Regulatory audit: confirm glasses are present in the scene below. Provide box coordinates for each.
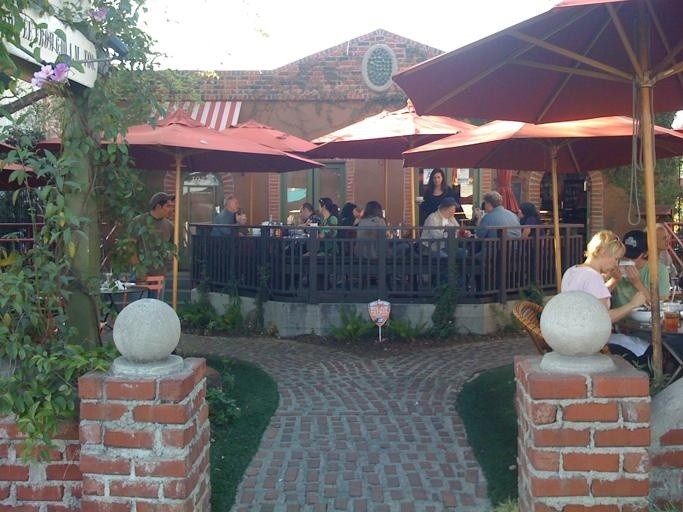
[660,235,670,241]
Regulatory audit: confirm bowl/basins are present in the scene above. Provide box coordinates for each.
[633,306,663,327]
[660,301,682,314]
[122,283,135,288]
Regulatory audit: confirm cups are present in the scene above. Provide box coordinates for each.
[458,228,466,239]
[618,261,634,280]
[663,305,679,335]
[671,276,679,291]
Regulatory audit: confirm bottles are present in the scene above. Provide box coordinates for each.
[268,214,276,236]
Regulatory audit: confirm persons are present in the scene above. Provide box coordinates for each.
[126,192,175,306]
[198,191,538,301]
[422,168,460,213]
[560,221,683,382]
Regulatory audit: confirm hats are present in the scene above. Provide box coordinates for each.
[148,192,175,210]
[621,230,647,258]
[437,197,456,208]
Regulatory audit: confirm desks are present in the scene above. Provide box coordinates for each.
[541,219,563,233]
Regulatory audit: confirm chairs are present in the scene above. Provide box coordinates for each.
[121,275,165,308]
[512,300,609,354]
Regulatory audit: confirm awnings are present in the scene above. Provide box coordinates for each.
[148,101,242,134]
